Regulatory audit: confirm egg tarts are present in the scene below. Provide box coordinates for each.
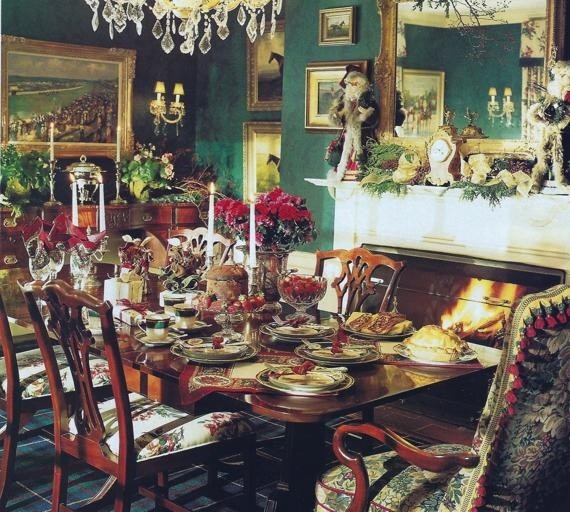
[278,372,335,392]
[275,326,317,335]
[313,347,360,360]
[189,345,246,357]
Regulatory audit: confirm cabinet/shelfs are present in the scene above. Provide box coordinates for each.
[334,182,569,318]
[0,202,209,253]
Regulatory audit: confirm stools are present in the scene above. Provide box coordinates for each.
[0,313,40,359]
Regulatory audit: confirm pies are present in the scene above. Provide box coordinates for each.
[346,312,412,334]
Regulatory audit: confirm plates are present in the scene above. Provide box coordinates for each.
[171,322,212,331]
[255,368,353,394]
[180,336,246,356]
[294,343,382,362]
[340,319,413,335]
[134,327,186,344]
[305,343,372,360]
[171,342,258,361]
[265,321,332,335]
[259,319,337,339]
[392,344,478,363]
[268,367,345,390]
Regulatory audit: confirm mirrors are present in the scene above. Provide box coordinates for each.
[373,1,569,164]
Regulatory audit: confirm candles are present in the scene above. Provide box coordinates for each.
[99,184,107,232]
[72,182,81,227]
[206,195,216,259]
[249,202,257,268]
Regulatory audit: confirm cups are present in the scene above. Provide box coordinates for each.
[164,294,185,313]
[48,251,63,278]
[28,256,50,306]
[174,304,198,328]
[138,314,169,339]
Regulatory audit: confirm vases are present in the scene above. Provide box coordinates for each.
[237,246,293,282]
[9,172,32,202]
[133,179,150,203]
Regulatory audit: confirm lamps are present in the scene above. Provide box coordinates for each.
[483,88,513,130]
[83,1,281,56]
[148,80,186,137]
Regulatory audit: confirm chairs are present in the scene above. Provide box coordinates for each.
[166,227,238,276]
[311,246,406,320]
[0,285,116,510]
[311,287,570,511]
[17,279,256,512]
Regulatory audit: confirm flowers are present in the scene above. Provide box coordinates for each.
[120,142,175,189]
[216,187,315,251]
[0,143,49,195]
[522,19,548,61]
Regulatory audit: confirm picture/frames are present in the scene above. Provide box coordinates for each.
[244,22,286,111]
[304,60,373,133]
[392,66,444,141]
[318,4,356,46]
[1,35,134,161]
[242,121,283,205]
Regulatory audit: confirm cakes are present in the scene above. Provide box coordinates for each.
[404,325,469,361]
[204,264,248,300]
